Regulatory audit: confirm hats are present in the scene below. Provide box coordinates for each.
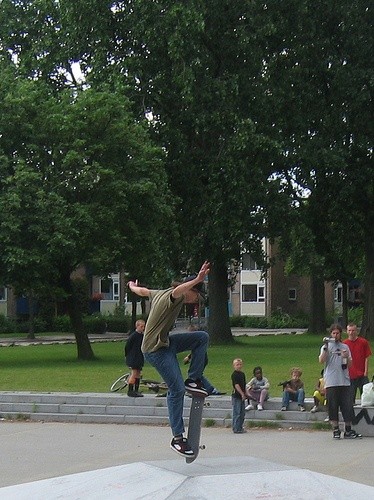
[182,277,206,300]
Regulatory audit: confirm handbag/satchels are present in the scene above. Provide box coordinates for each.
[360,382,374,407]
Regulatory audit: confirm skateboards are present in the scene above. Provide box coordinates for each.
[186,395,210,464]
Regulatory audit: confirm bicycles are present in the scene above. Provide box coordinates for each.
[109,372,170,394]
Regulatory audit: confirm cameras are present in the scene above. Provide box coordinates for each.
[324,337,336,343]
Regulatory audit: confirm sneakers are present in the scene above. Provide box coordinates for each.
[185,378,209,398]
[323,416,330,422]
[241,427,245,430]
[257,404,263,411]
[234,429,246,433]
[244,405,254,411]
[333,428,341,440]
[281,406,286,411]
[310,405,319,413]
[206,386,217,395]
[170,438,195,458]
[135,391,143,397]
[344,430,362,439]
[297,406,306,412]
[127,391,137,397]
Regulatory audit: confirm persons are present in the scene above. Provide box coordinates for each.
[125,320,145,397]
[182,351,217,395]
[232,358,248,433]
[126,260,211,457]
[244,366,270,411]
[281,367,306,411]
[318,323,363,439]
[361,376,374,407]
[342,322,372,406]
[310,369,327,412]
